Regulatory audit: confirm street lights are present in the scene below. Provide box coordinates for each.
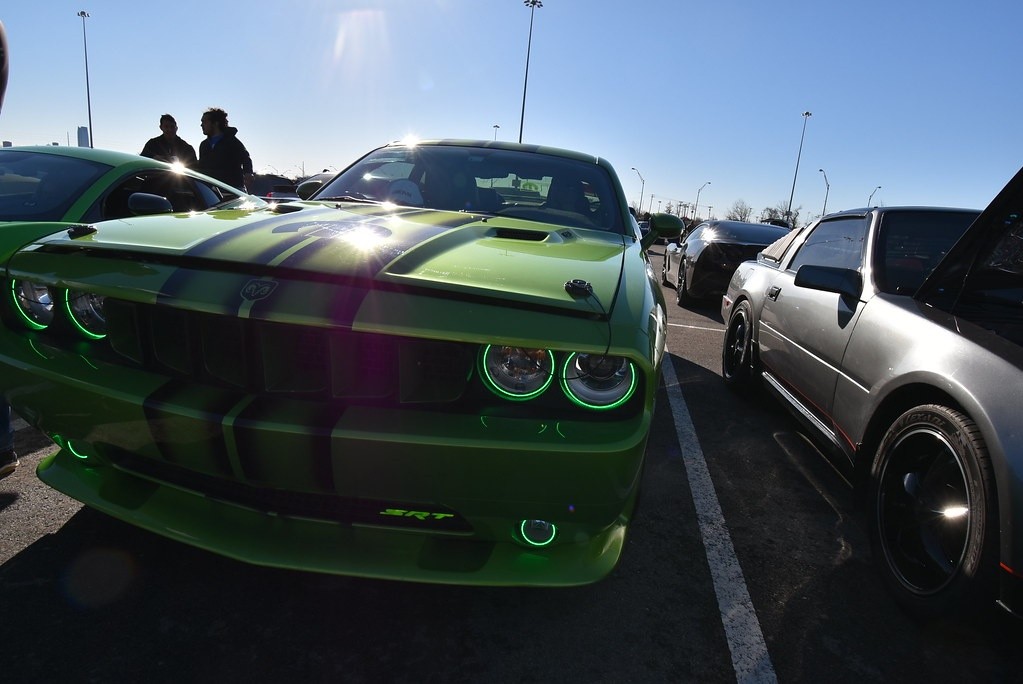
[694,181,711,221]
[519,0,543,143]
[819,169,829,215]
[78,11,94,149]
[632,167,644,215]
[868,186,881,207]
[787,112,812,220]
[491,125,499,188]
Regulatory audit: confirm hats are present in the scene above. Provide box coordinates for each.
[387,179,423,206]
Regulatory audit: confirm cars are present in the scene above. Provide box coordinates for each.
[0,148,269,267]
[0,141,667,594]
[663,220,792,307]
[720,167,1023,618]
[267,173,336,197]
[638,221,649,235]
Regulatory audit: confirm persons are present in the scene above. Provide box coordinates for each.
[199,108,255,194]
[139,114,198,171]
[0,19,18,478]
[686,221,695,233]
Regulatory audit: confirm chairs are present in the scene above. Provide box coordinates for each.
[419,173,583,211]
